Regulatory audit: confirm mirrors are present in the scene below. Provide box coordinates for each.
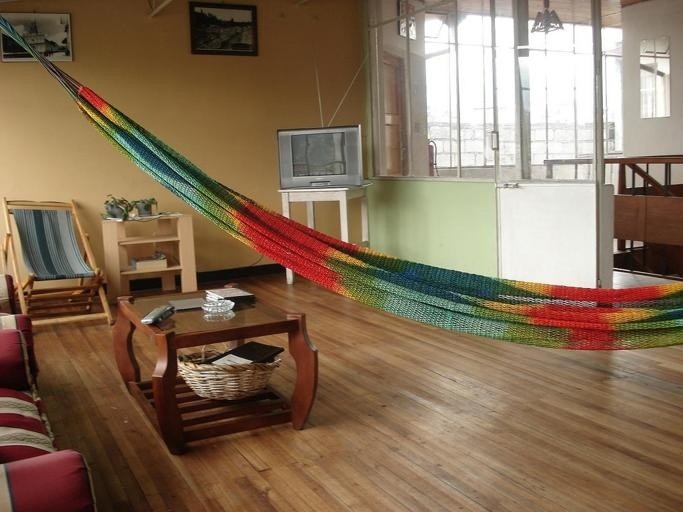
[640,37,671,118]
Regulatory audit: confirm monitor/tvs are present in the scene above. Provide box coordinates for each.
[276,124,364,190]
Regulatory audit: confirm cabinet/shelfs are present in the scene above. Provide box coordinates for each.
[101,212,199,304]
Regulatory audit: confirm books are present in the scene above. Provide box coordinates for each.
[204,287,255,303]
[203,339,283,366]
[129,250,168,273]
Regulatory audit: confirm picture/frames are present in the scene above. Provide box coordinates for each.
[0,12,73,62]
[396,0,417,41]
[189,0,259,57]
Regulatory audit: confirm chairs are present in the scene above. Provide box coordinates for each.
[1,197,115,334]
[0,312,99,511]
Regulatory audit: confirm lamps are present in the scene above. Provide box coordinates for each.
[530,0,565,36]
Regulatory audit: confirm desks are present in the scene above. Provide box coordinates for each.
[276,181,375,285]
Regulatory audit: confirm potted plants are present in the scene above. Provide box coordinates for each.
[103,193,134,220]
[128,196,160,217]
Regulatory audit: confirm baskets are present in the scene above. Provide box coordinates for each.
[177,341,282,401]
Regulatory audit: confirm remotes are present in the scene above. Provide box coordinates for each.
[140,305,173,325]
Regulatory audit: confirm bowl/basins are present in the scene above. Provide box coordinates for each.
[203,315,235,321]
[202,301,234,313]
